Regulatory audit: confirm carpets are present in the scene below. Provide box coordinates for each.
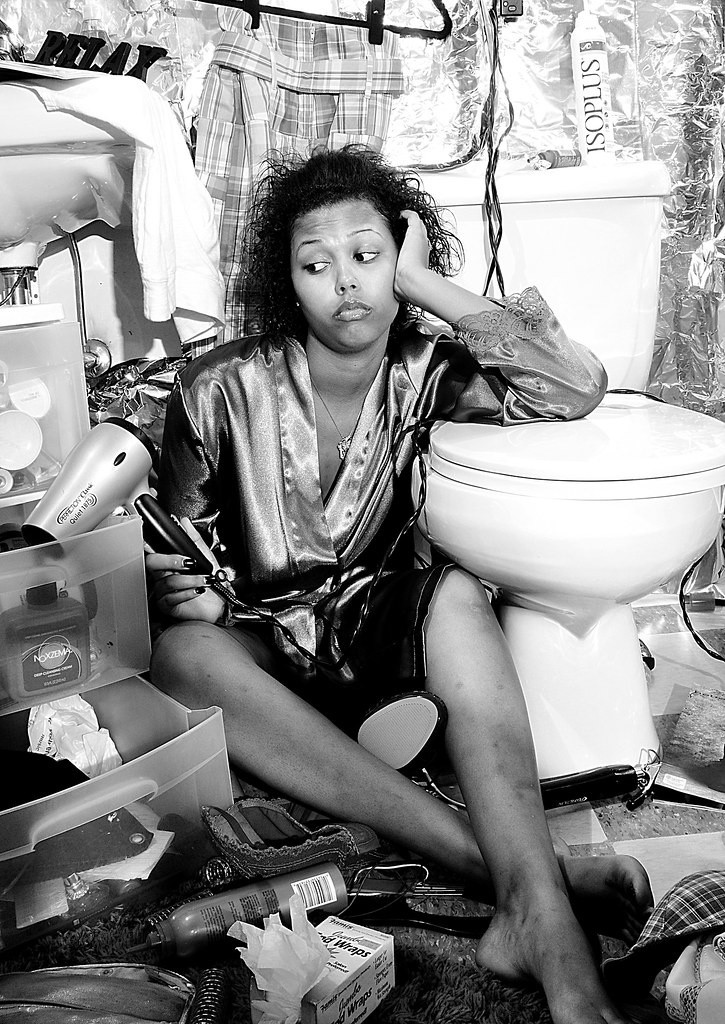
[1,885,670,1024]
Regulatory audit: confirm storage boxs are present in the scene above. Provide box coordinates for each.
[250,915,395,1024]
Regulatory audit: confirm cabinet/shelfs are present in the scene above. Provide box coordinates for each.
[0,302,236,953]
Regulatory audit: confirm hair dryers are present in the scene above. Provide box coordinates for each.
[21,417,213,575]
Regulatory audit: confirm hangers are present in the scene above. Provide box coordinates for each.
[197,0,453,47]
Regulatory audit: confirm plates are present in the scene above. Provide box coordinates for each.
[0,410,43,470]
[7,378,51,418]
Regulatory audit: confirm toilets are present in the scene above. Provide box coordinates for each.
[412,394,725,769]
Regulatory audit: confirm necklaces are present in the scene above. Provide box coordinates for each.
[310,377,362,460]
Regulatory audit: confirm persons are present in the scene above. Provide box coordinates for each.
[146,145,654,1024]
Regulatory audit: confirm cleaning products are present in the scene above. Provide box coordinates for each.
[570,10,614,160]
